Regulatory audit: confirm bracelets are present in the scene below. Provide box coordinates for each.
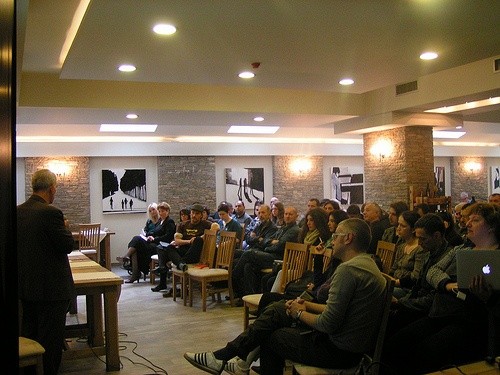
[296,309,303,319]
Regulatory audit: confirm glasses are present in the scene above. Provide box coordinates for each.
[331,233,347,240]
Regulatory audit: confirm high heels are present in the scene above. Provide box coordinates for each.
[125,272,142,283]
[115,256,131,264]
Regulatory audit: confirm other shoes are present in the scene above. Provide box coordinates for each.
[251,366,262,374]
[150,285,167,292]
[162,287,181,298]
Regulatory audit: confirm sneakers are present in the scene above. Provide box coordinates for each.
[224,360,250,375]
[184,350,226,375]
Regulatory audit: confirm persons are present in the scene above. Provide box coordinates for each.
[205,196,500,306]
[116,202,174,285]
[384,200,499,375]
[15,168,77,373]
[184,218,387,375]
[178,208,191,222]
[166,203,214,273]
[389,214,460,329]
[149,208,191,292]
[251,242,343,375]
[382,210,426,295]
[121,203,161,269]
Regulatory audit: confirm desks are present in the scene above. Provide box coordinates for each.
[65,250,124,372]
[72,232,115,271]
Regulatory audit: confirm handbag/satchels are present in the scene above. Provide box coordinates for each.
[284,270,318,297]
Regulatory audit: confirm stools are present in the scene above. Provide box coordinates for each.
[18,336,46,375]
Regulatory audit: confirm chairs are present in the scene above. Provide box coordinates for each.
[78,224,101,263]
[242,240,395,375]
[151,230,236,312]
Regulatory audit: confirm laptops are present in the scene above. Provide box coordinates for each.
[455,249,500,291]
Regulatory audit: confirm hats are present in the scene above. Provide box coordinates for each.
[217,200,230,213]
[190,204,204,212]
